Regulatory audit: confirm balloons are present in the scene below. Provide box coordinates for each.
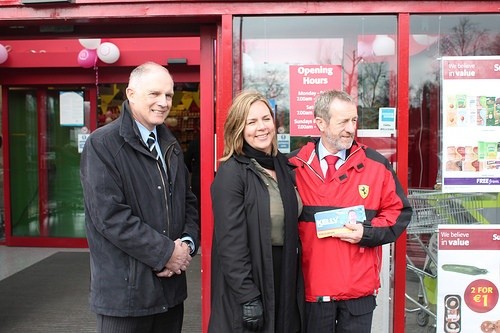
[78,39,101,51]
[0,44,8,64]
[78,49,97,68]
[98,42,120,64]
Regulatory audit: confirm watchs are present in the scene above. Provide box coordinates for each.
[182,240,194,255]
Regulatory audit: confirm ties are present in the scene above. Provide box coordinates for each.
[324,155,339,182]
[147,132,171,211]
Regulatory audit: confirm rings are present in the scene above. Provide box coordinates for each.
[169,272,173,274]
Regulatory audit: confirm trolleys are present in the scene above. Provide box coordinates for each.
[406,188,485,332]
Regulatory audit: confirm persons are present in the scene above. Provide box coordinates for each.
[207,92,303,333]
[285,91,413,333]
[342,210,361,227]
[79,62,200,333]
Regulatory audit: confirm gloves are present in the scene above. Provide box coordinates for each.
[242,298,264,333]
